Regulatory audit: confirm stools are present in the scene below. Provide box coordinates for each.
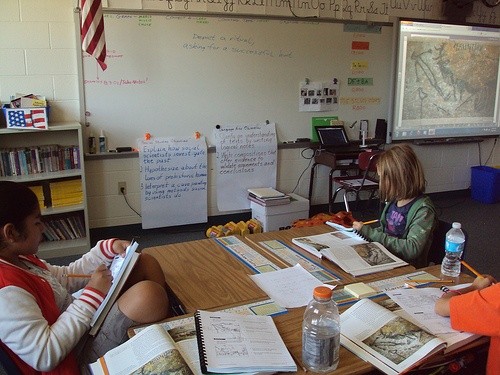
[251,193,309,232]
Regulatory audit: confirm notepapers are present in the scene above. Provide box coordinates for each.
[344,282,377,299]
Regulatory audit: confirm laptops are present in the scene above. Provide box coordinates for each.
[315,125,365,154]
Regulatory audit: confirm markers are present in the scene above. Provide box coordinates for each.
[282,141,297,143]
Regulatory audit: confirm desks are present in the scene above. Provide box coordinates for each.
[128,220,491,375]
[308,145,386,214]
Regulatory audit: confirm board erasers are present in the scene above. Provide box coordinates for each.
[297,138,310,142]
[116,147,132,152]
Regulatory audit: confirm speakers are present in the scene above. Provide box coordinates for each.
[376,119,387,144]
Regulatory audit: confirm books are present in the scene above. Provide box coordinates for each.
[30,180,82,209]
[196,309,297,375]
[248,188,291,206]
[89,317,200,375]
[72,238,141,336]
[335,282,500,375]
[0,144,80,177]
[41,215,85,240]
[293,231,409,278]
[10,94,46,109]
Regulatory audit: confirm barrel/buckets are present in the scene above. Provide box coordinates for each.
[470,165,500,204]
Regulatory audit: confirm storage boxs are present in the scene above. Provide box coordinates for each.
[2,104,50,128]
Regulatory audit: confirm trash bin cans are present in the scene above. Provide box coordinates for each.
[470,166,500,203]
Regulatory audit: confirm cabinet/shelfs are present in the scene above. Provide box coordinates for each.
[0,121,90,259]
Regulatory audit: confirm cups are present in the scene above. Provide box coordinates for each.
[441,222,466,277]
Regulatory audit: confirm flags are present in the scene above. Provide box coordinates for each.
[78,0,108,71]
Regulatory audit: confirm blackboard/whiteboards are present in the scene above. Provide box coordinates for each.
[74,5,395,160]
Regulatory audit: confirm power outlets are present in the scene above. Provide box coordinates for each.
[118,181,127,196]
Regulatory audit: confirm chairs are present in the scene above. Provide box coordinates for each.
[432,220,467,265]
[331,151,384,220]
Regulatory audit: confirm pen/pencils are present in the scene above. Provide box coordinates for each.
[364,219,379,225]
[458,257,485,279]
[405,280,454,282]
[62,274,91,277]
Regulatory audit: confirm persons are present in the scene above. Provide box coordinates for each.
[352,143,436,263]
[434,274,500,375]
[0,181,169,375]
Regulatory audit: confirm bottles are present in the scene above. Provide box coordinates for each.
[301,285,341,374]
[88,133,96,153]
[99,128,106,153]
